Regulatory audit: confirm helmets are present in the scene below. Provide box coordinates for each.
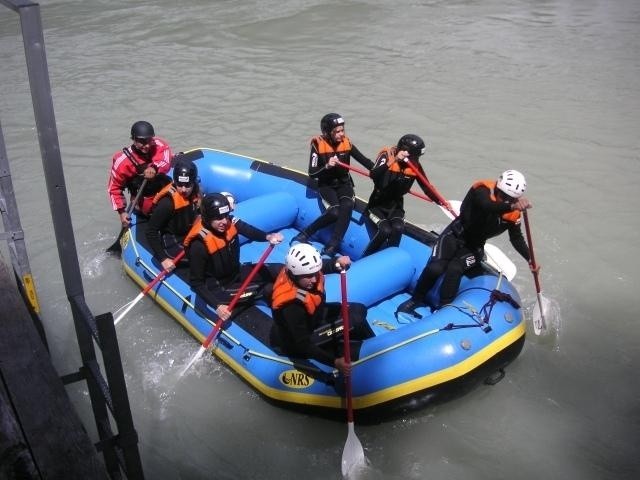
[397,134,426,157]
[496,169,527,199]
[131,121,156,139]
[200,193,232,219]
[286,244,323,276]
[173,159,198,186]
[321,113,345,132]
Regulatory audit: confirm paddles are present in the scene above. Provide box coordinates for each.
[334,155,463,220]
[112,249,184,326]
[182,238,277,375]
[105,174,148,257]
[404,157,517,282]
[335,263,367,479]
[523,207,551,336]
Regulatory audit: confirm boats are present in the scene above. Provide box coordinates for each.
[123,146,525,429]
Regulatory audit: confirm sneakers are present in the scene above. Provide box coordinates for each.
[397,297,424,314]
[288,230,313,247]
[321,247,336,259]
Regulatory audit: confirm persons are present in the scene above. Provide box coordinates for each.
[146,160,205,274]
[272,243,375,385]
[189,192,285,322]
[107,120,173,229]
[289,112,375,258]
[394,170,541,313]
[362,133,453,256]
